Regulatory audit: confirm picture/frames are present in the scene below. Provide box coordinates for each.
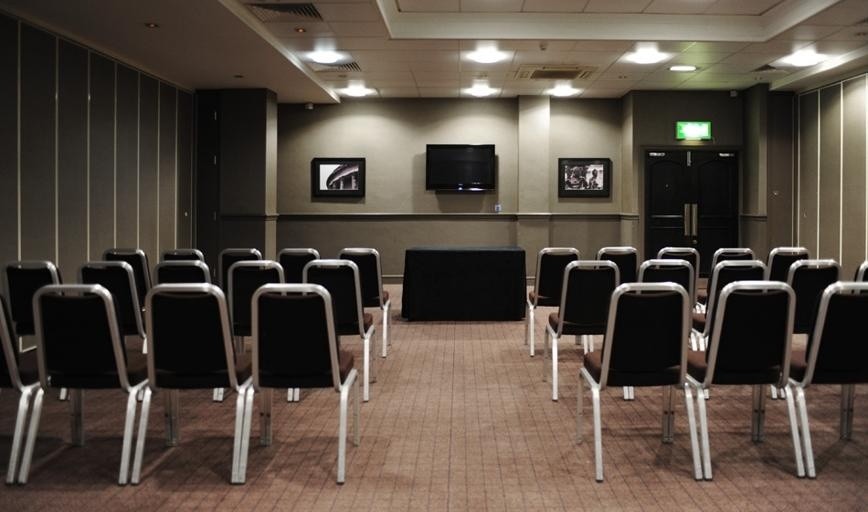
[557,157,611,198]
[311,156,366,199]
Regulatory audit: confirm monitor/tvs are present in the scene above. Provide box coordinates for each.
[426,144,495,191]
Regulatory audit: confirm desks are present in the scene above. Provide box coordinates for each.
[401,244,527,321]
